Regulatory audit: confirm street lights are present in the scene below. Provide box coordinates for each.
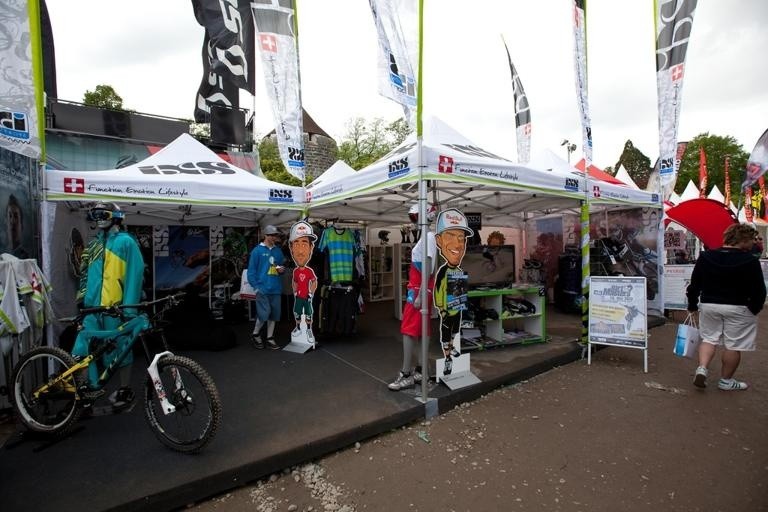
[559,139,576,168]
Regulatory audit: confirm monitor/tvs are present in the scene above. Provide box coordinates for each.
[461,245,515,290]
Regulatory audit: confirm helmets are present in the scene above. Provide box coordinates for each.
[378,231,390,242]
[95,202,120,223]
[522,259,543,269]
[407,203,435,222]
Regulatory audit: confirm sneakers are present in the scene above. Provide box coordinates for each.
[717,379,747,391]
[443,359,451,374]
[250,334,264,350]
[306,329,313,343]
[112,386,136,413]
[413,366,429,384]
[387,370,414,391]
[449,347,459,357]
[692,368,707,388]
[265,337,280,350]
[291,327,301,336]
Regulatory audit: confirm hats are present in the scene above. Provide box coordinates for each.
[290,222,315,242]
[263,225,280,234]
[436,209,474,237]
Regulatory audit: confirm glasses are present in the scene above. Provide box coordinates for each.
[409,214,417,223]
[92,210,124,220]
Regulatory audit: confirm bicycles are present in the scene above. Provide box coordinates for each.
[8,292,223,458]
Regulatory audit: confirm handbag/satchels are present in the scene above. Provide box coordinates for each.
[672,313,699,359]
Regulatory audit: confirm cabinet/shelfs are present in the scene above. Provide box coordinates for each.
[363,245,395,302]
[453,287,546,354]
[395,243,415,320]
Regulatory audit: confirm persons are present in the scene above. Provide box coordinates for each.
[530,232,560,305]
[288,221,318,343]
[487,231,506,245]
[1,193,30,259]
[482,244,493,262]
[685,223,767,390]
[432,207,475,374]
[72,201,147,411]
[247,225,288,349]
[387,204,435,392]
